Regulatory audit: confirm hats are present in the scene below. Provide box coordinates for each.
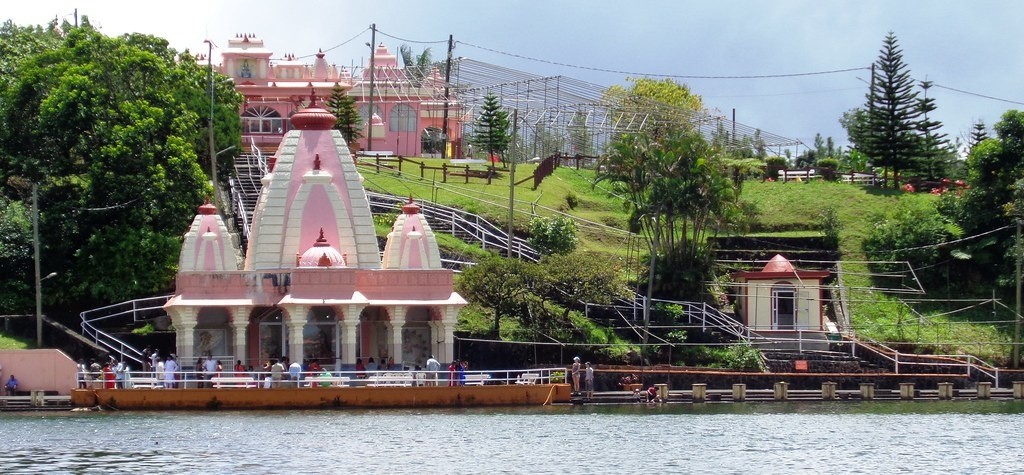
[457,359,461,362]
[573,357,580,360]
[90,363,101,371]
[636,389,639,392]
[585,362,590,364]
[453,360,457,363]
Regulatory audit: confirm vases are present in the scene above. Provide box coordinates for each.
[623,384,644,390]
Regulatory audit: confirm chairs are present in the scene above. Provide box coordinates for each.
[383,374,413,387]
[416,373,426,386]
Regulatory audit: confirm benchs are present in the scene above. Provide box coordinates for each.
[211,377,256,389]
[463,375,491,386]
[10,391,58,396]
[367,376,412,388]
[515,373,539,385]
[115,378,163,389]
[304,377,350,388]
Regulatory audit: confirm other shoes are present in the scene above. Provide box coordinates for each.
[646,400,649,403]
[4,392,8,396]
[650,400,655,403]
[572,392,580,396]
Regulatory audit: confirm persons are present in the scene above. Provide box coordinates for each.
[196,354,223,388]
[585,362,594,398]
[426,355,440,386]
[356,357,386,378]
[448,359,465,386]
[77,358,131,390]
[235,357,332,388]
[4,375,18,396]
[572,356,581,394]
[142,345,180,388]
[646,387,659,402]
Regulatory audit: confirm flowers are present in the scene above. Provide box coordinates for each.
[619,374,641,386]
